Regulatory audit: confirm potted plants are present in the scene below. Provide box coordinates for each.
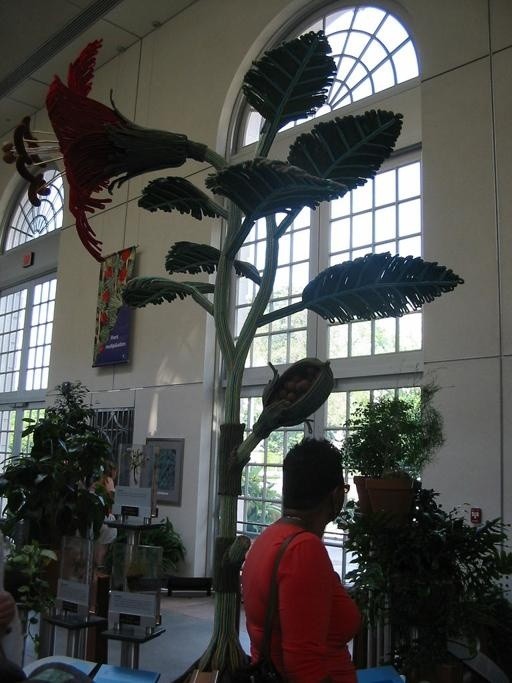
[366,391,447,526]
[338,519,510,683]
[105,516,186,591]
[339,403,372,512]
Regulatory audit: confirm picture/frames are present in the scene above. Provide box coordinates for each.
[140,438,184,506]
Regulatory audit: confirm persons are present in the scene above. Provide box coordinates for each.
[241,439,363,683]
[89,461,118,570]
[0,532,29,682]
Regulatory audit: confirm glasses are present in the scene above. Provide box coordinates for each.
[339,484,349,493]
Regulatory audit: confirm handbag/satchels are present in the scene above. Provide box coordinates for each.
[235,656,281,683]
